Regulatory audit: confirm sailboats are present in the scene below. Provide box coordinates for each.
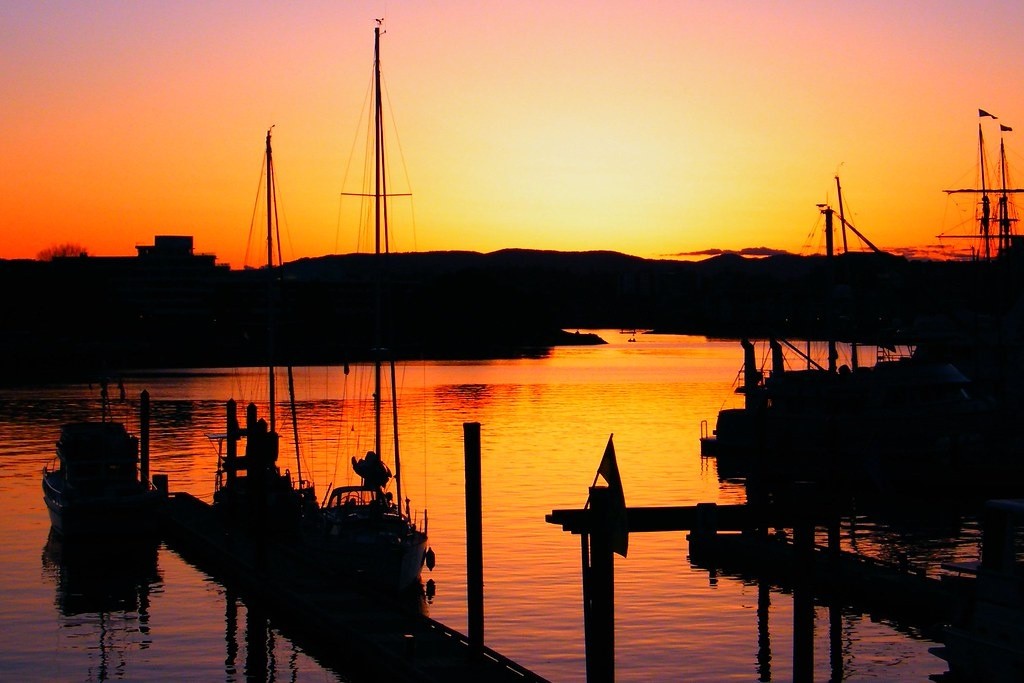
[297,18,430,599]
[204,122,318,546]
[697,107,1024,489]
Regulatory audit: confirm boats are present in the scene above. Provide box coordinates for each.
[40,371,161,545]
